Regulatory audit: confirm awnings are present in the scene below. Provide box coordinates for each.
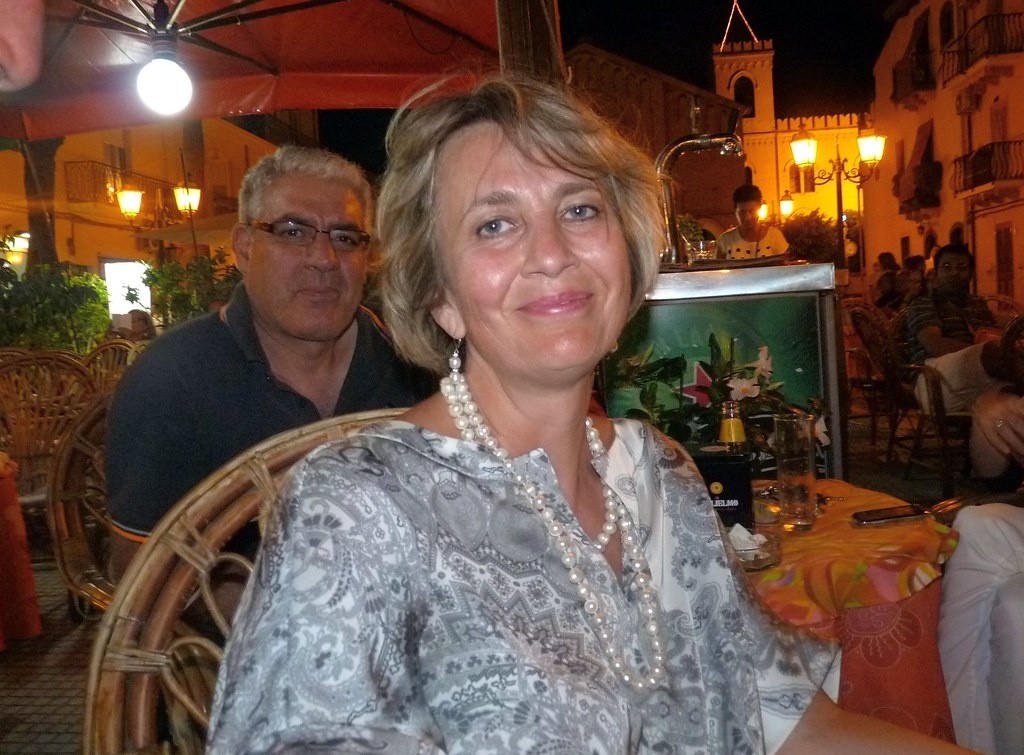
[898,7,931,99]
[899,120,934,203]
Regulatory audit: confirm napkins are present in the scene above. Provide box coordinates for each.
[728,522,771,562]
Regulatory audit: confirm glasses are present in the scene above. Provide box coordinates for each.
[248,218,372,253]
[937,263,972,272]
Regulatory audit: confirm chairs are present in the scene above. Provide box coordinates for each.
[840,292,1024,498]
[45,389,219,755]
[84,405,413,755]
[0,339,152,554]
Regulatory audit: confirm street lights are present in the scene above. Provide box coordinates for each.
[115,166,203,331]
[788,111,888,287]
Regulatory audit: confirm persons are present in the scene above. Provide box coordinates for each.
[871,252,936,311]
[206,66,976,754]
[903,246,1024,414]
[209,300,227,310]
[713,184,790,262]
[967,392,1024,494]
[105,145,439,647]
[118,310,156,341]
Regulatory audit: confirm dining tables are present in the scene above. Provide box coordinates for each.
[724,478,960,746]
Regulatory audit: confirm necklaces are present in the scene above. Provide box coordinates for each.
[439,371,666,696]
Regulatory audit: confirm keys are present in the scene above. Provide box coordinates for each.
[817,493,846,503]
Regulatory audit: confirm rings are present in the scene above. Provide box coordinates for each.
[994,420,1004,430]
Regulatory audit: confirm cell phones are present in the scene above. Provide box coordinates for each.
[853,505,925,526]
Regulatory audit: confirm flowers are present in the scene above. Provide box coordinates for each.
[694,332,786,406]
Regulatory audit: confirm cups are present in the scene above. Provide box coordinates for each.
[686,240,718,262]
[774,413,816,532]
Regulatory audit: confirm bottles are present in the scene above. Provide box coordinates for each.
[719,400,749,453]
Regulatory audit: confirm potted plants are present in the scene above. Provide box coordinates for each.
[676,215,718,260]
